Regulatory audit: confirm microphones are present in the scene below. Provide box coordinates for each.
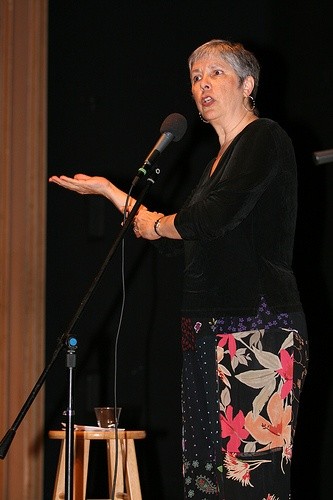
[132,113,188,184]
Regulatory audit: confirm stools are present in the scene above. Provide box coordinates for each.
[48,430,146,500]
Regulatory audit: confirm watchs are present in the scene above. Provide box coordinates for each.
[155,217,164,238]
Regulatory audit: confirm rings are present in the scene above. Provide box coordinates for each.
[133,227,139,232]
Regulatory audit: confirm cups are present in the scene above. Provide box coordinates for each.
[93,407,121,429]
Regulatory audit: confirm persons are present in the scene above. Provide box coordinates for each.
[49,40,309,500]
